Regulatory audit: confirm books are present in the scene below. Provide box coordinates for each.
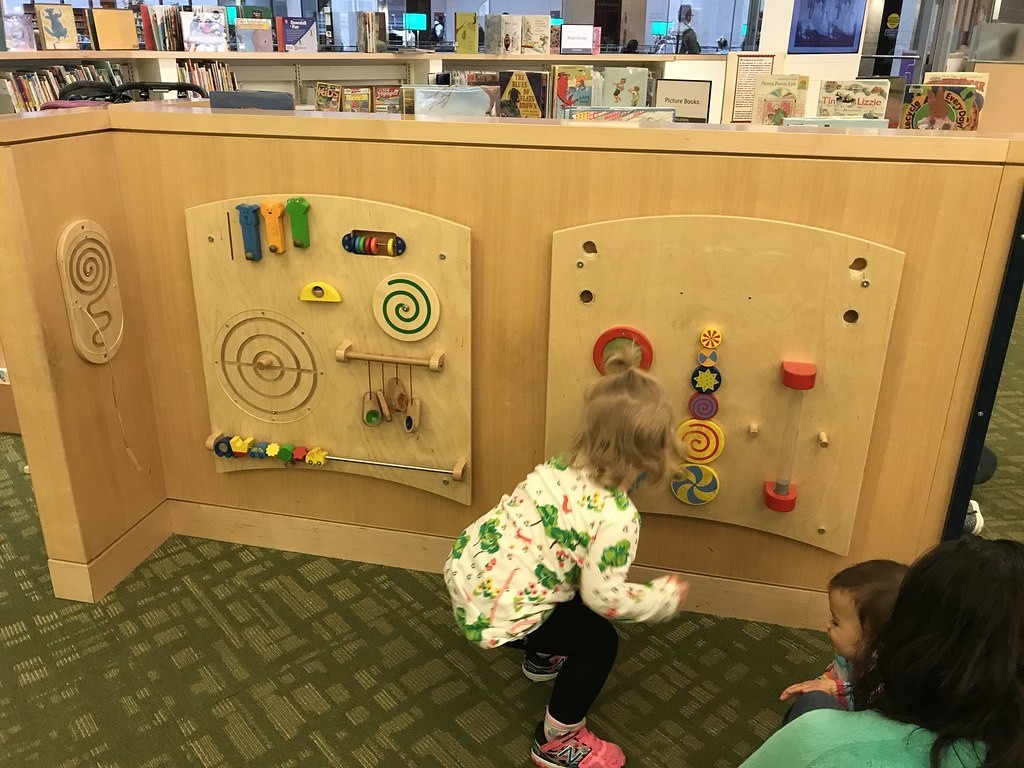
[1,1,1022,129]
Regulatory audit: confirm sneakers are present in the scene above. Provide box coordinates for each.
[522,647,569,685]
[529,719,626,768]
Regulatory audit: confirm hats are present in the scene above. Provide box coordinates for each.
[678,4,694,18]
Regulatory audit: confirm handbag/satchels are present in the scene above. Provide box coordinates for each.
[427,23,443,44]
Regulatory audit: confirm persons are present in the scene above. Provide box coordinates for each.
[442,333,691,768]
[779,558,911,711]
[738,534,1024,768]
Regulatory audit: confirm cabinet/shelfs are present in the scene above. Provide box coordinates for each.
[0,45,733,117]
